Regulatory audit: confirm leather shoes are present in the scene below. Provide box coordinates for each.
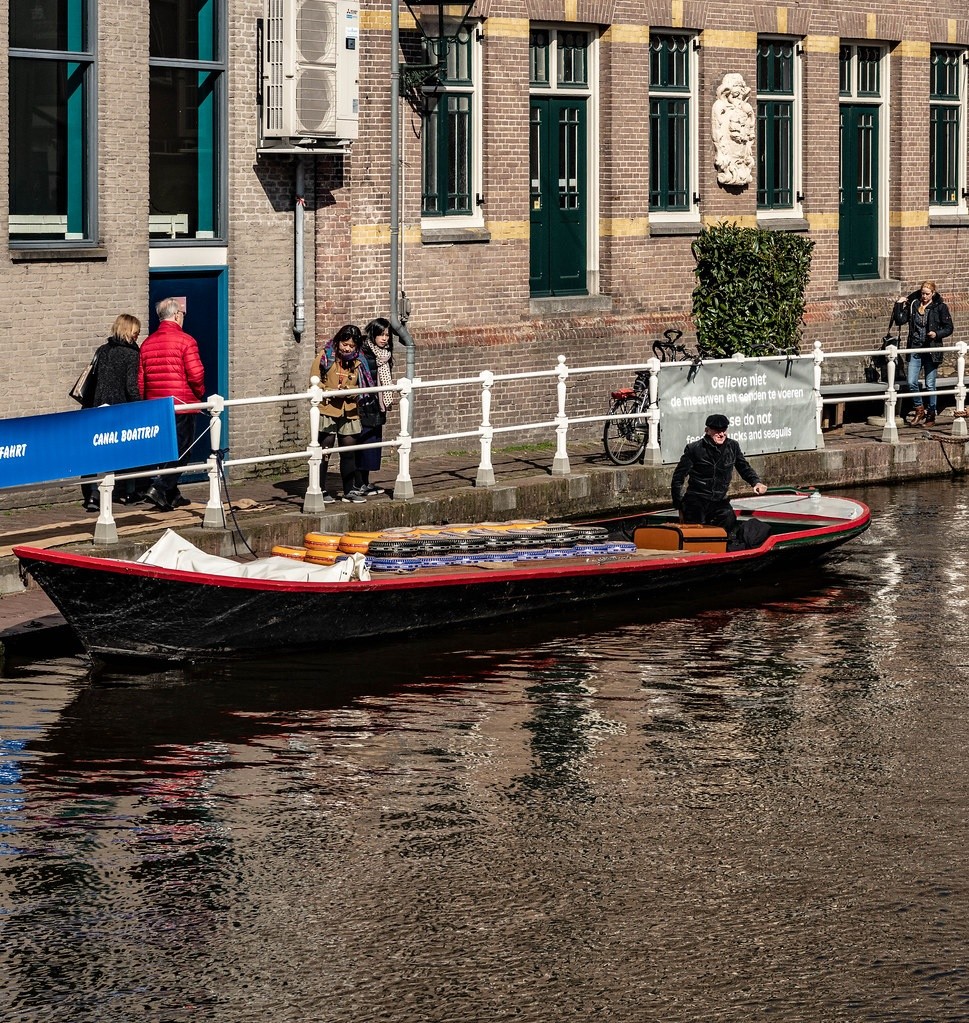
[125,492,147,505]
[166,495,191,507]
[87,496,100,509]
[146,486,173,510]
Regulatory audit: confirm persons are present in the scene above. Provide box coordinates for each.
[86,314,147,509]
[894,281,954,429]
[671,414,768,552]
[308,317,394,503]
[137,297,205,513]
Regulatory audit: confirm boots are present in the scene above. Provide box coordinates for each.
[911,404,925,425]
[921,410,936,427]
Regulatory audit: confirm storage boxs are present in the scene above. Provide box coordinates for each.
[631,524,729,552]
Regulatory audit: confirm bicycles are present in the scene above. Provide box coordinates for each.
[602,329,796,466]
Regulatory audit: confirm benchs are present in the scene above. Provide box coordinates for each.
[820,377,969,429]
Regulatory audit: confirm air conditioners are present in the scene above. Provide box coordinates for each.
[264,0,359,140]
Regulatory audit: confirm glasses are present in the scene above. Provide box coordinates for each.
[177,310,185,314]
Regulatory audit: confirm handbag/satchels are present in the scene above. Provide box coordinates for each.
[880,334,901,349]
[69,345,104,406]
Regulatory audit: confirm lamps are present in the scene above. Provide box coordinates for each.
[396,0,477,96]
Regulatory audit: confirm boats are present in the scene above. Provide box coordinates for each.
[11,487,873,671]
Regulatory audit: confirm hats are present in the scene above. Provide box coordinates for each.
[705,414,729,430]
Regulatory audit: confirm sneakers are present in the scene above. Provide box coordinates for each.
[323,490,336,504]
[341,492,367,503]
[368,483,385,493]
[350,484,378,495]
